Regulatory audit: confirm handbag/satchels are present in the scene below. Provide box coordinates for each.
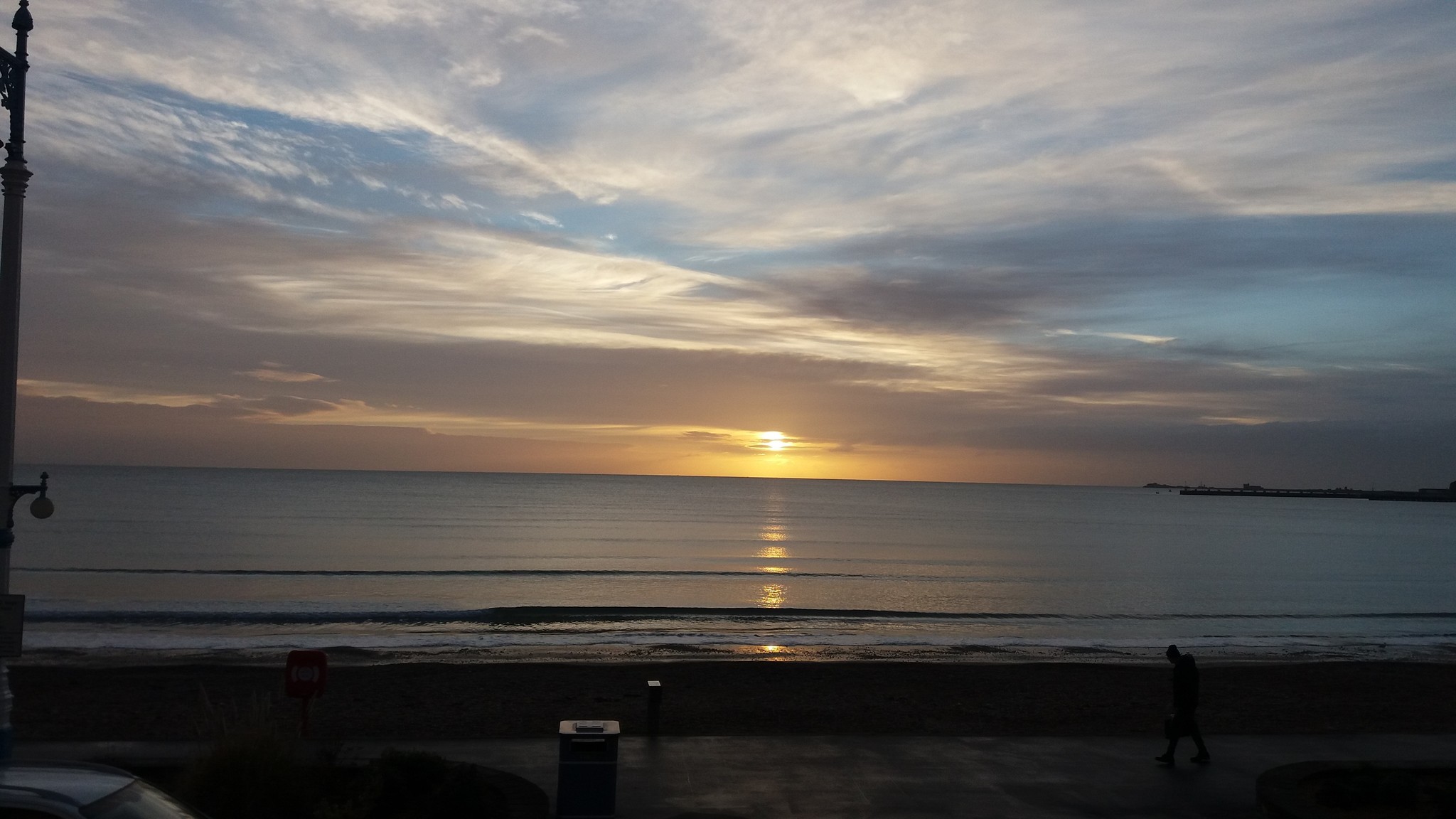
[1163,719,1175,739]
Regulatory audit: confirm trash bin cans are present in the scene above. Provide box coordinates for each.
[0,594,25,658]
[557,720,620,819]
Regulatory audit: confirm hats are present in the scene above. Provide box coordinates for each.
[1166,645,1180,658]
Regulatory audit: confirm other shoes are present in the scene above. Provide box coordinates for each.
[1190,754,1210,762]
[1154,754,1174,763]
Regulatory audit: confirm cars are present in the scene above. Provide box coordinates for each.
[0,760,198,818]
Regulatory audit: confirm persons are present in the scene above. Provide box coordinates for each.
[1155,644,1210,763]
